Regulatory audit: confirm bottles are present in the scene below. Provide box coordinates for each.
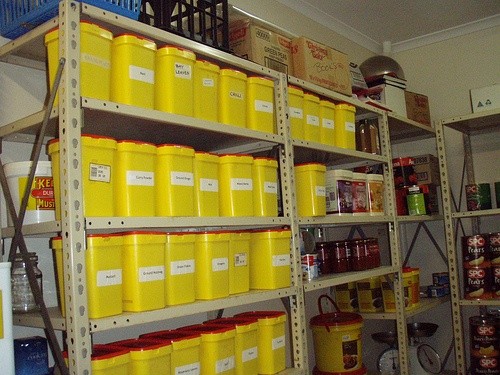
[10,250,44,313]
[318,237,380,275]
[408,186,426,215]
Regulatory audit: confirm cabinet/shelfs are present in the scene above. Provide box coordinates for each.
[0,0,435,375]
[434,108,500,375]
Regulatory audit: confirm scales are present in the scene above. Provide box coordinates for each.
[408,322,448,375]
[372,331,399,375]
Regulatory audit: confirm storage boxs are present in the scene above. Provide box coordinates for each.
[336,266,449,312]
[469,83,500,113]
[411,153,440,186]
[404,90,430,124]
[292,33,352,97]
[138,0,234,54]
[369,83,407,118]
[155,44,197,117]
[325,170,354,216]
[335,101,357,150]
[218,19,294,76]
[170,57,336,375]
[44,18,170,375]
[349,61,369,96]
[352,173,385,217]
[0,0,141,40]
[421,184,439,214]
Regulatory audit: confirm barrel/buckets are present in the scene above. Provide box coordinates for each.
[309,295,366,375]
[3,161,58,224]
